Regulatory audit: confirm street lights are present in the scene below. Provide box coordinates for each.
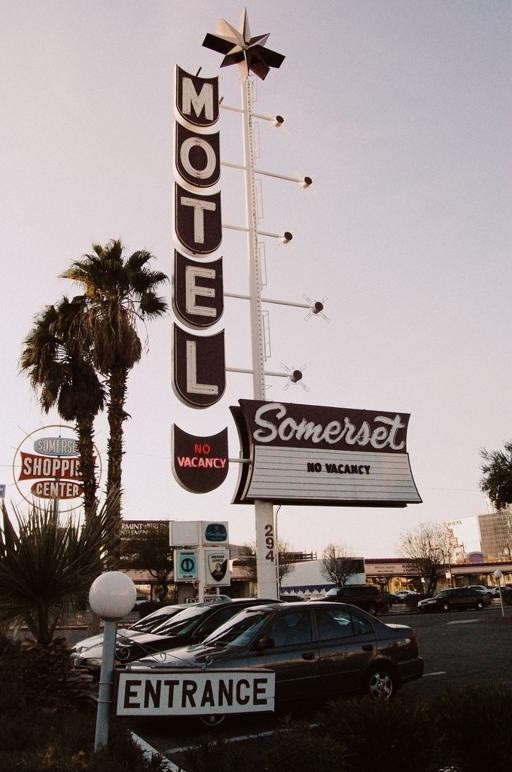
[494,569,504,616]
[88,571,137,752]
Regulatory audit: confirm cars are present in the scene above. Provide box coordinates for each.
[418,584,512,613]
